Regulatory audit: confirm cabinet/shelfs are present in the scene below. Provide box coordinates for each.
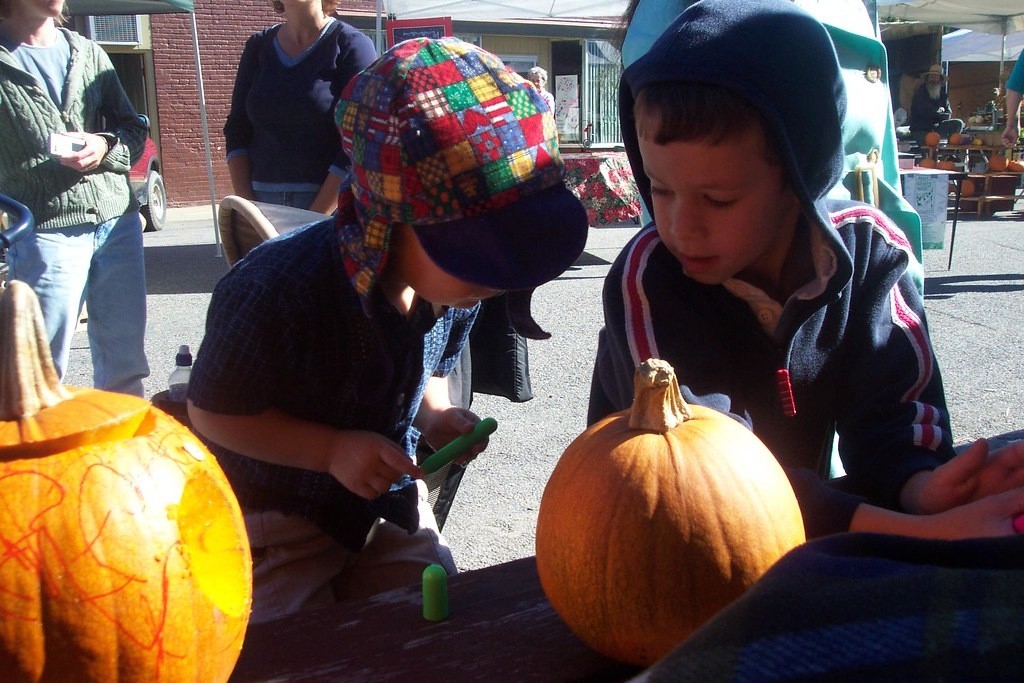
[920,115,1024,222]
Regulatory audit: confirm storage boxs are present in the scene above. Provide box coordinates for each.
[976,131,1003,146]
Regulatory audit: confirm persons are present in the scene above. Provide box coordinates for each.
[0,2,153,410]
[586,0,1024,549]
[524,65,555,122]
[1001,45,1024,150]
[222,0,380,216]
[184,35,590,635]
[618,0,924,303]
[909,64,964,159]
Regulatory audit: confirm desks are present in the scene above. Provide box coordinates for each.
[560,153,642,227]
[228,440,979,683]
[899,165,970,271]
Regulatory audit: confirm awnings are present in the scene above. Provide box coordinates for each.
[383,2,632,38]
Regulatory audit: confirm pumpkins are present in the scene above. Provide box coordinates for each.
[919,129,1024,197]
[532,356,805,667]
[0,278,253,683]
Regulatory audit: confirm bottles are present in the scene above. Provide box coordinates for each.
[168,345,192,401]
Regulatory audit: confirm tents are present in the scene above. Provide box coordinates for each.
[875,0,1024,72]
[67,0,224,260]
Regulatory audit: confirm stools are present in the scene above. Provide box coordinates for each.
[959,175,1017,212]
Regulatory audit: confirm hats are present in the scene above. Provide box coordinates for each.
[332,36,590,303]
[920,64,950,83]
